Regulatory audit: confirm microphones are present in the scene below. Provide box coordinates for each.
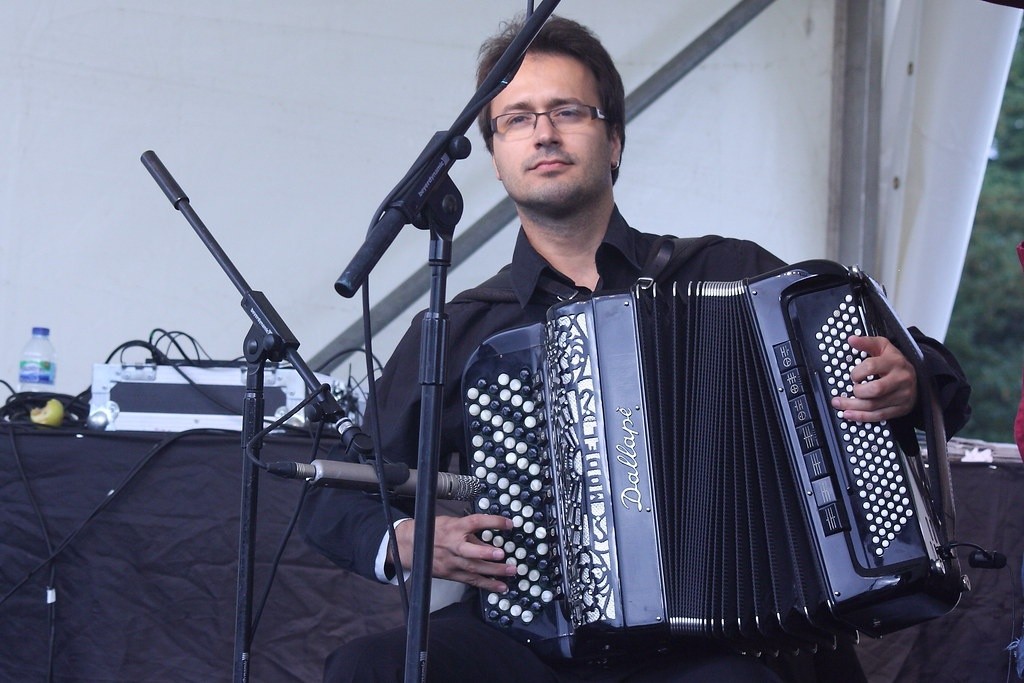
[265,458,481,503]
[944,542,1008,570]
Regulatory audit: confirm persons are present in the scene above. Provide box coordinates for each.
[297,14,973,683]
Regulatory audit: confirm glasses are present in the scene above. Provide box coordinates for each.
[491,103,610,137]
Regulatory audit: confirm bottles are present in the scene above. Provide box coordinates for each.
[18,327,56,393]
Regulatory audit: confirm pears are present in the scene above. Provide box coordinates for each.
[30,398,63,426]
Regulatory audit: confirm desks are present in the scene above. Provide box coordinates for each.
[0,421,1024,683]
